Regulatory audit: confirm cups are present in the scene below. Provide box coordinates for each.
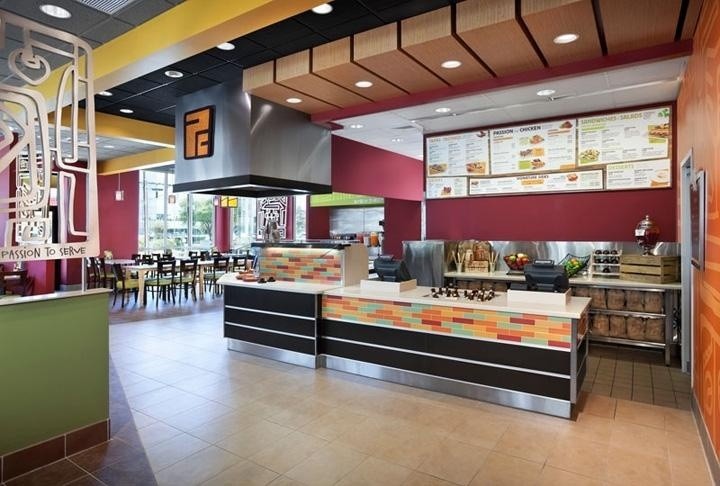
[456,263,463,271]
[490,263,495,274]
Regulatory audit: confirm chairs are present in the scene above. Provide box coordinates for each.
[0,270,28,296]
[89,248,259,308]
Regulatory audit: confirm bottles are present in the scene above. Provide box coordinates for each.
[357,232,379,247]
[635,214,659,256]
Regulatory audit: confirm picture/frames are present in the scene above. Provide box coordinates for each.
[689,171,706,272]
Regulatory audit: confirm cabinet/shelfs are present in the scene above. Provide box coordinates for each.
[590,248,623,279]
[451,276,674,367]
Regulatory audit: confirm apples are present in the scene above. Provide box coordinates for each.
[505,256,528,270]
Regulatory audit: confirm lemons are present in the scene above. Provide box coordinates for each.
[563,258,584,275]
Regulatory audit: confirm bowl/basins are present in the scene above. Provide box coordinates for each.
[504,257,532,271]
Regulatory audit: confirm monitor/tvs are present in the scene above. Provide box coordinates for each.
[524,263,569,293]
